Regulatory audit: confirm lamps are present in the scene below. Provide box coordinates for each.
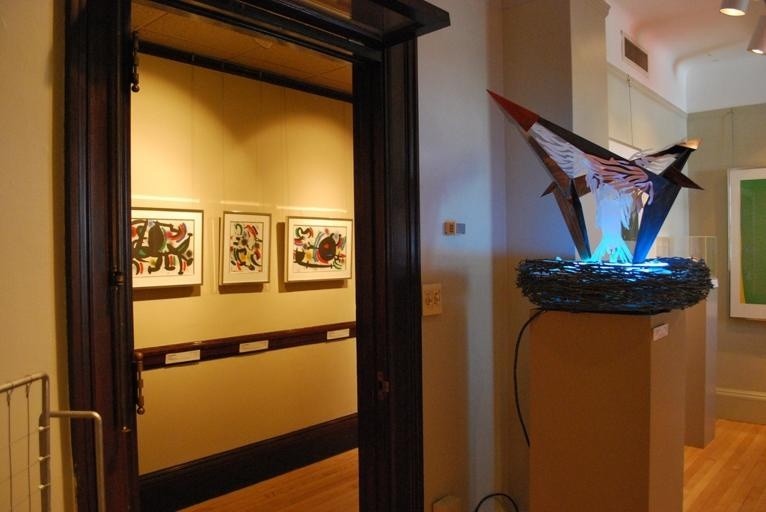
[746,13,765,58]
[716,0,751,19]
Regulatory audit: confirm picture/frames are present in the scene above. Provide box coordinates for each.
[725,167,766,324]
[217,209,276,289]
[128,205,209,291]
[282,215,354,288]
